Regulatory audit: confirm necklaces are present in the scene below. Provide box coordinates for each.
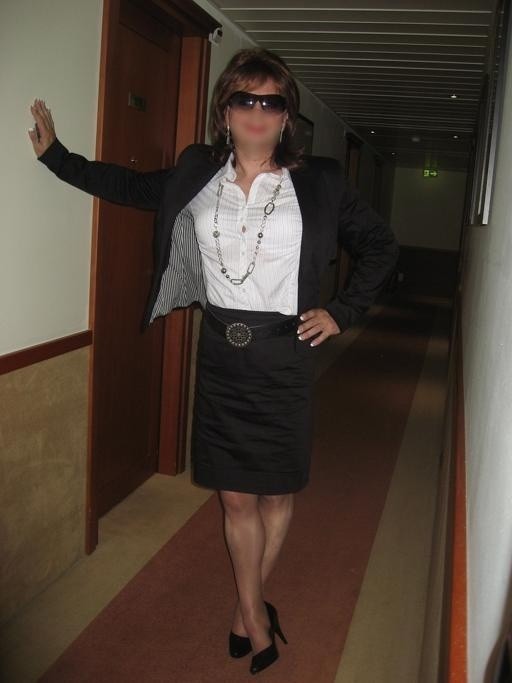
[213,166,284,285]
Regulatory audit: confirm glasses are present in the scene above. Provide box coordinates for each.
[227,91,288,116]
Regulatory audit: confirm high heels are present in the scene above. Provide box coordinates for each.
[229,600,289,675]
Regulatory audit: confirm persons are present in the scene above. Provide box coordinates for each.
[28,46,400,674]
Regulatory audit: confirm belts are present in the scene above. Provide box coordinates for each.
[205,304,297,349]
